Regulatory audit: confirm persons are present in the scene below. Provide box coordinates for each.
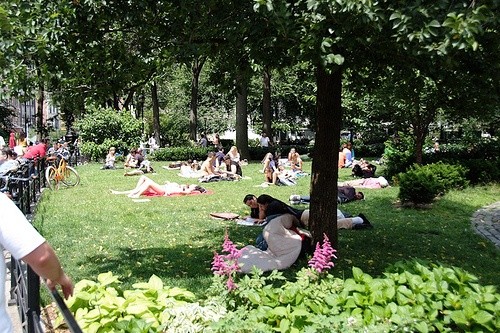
[259,132,274,147]
[433,140,441,152]
[0,190,74,333]
[0,130,80,179]
[101,131,242,199]
[261,147,308,187]
[235,193,372,271]
[338,142,389,188]
[289,185,364,205]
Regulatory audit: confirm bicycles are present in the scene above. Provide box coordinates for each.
[45,139,80,191]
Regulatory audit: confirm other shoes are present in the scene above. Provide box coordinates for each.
[289,195,301,201]
[290,201,300,205]
[358,213,373,228]
